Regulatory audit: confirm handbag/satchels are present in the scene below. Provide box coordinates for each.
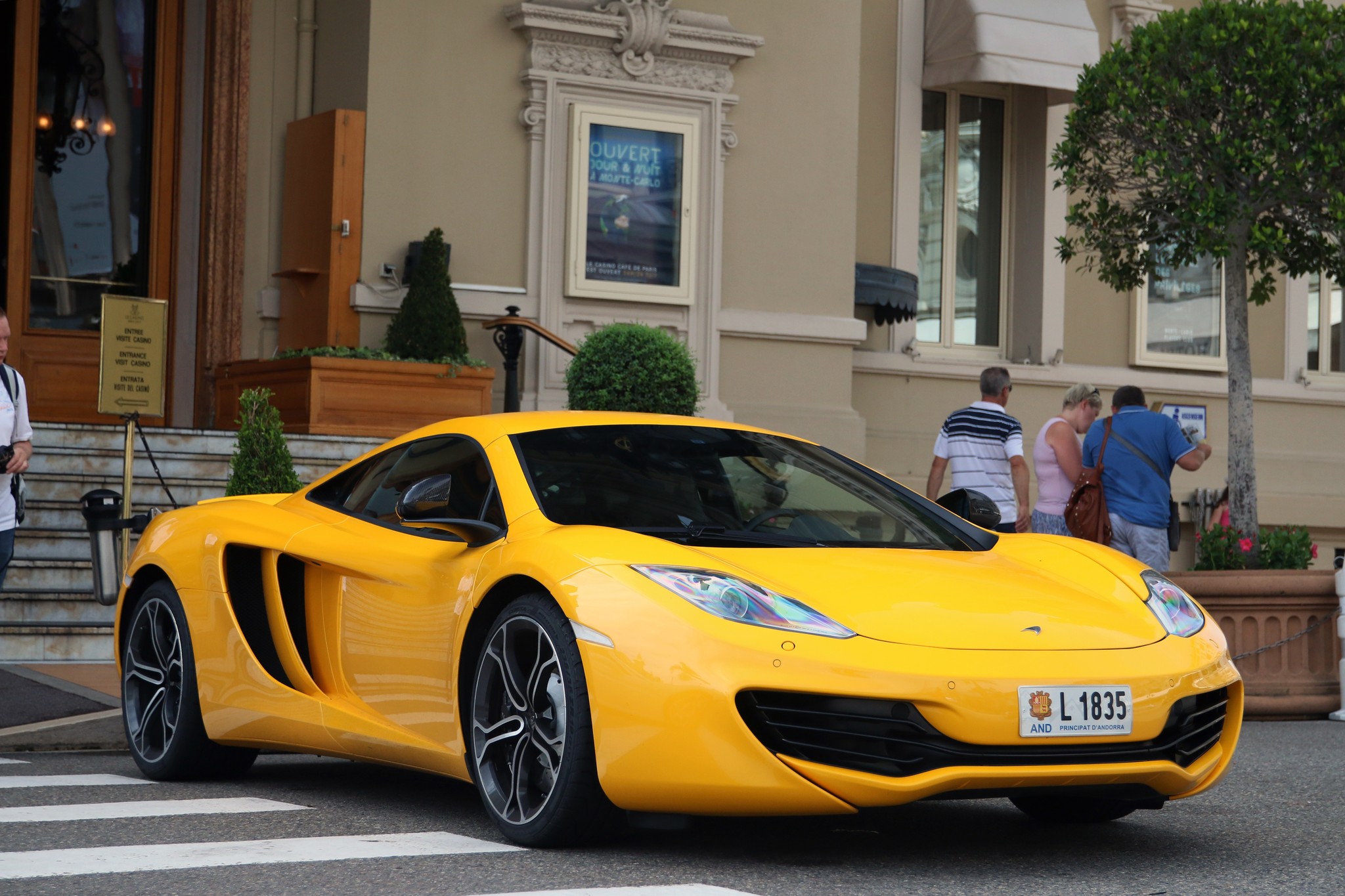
[1168,500,1182,552]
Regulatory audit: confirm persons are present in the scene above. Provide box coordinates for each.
[0,310,34,595]
[926,366,1030,535]
[1032,384,1103,538]
[1081,385,1212,573]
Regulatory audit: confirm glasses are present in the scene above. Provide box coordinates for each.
[1089,387,1100,396]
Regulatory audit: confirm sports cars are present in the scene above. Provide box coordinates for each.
[111,407,1248,845]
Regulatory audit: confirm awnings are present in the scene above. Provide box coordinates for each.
[854,262,919,326]
[923,1,1102,106]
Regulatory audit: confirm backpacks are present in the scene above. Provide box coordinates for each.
[1064,415,1114,547]
